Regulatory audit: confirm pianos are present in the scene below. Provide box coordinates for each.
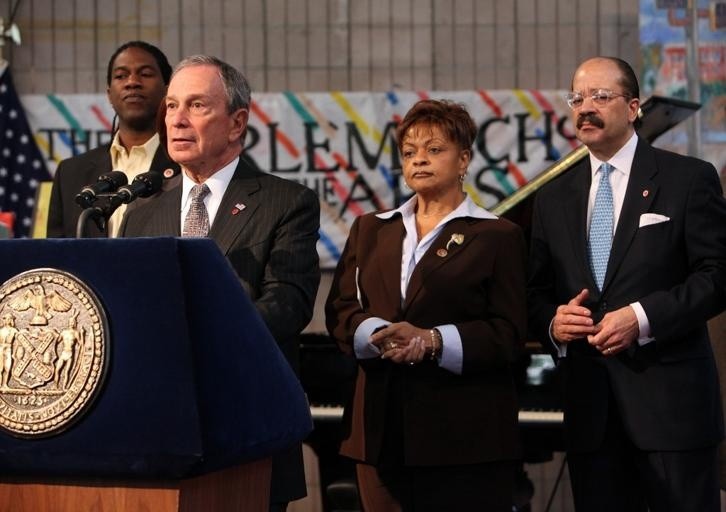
[298,97,702,510]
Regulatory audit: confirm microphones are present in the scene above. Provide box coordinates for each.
[105,170,162,211]
[74,171,127,207]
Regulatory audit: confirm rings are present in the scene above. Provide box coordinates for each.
[387,340,398,349]
[606,346,612,358]
[408,360,416,367]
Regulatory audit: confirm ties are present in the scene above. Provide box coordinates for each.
[587,163,615,294]
[183,185,210,240]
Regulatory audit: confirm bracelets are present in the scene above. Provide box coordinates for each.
[427,329,438,362]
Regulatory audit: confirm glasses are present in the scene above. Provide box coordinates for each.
[564,88,632,110]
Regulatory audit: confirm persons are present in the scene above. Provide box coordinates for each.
[55,313,86,391]
[48,41,174,237]
[1,310,24,393]
[321,97,525,509]
[523,56,723,512]
[108,53,318,511]
